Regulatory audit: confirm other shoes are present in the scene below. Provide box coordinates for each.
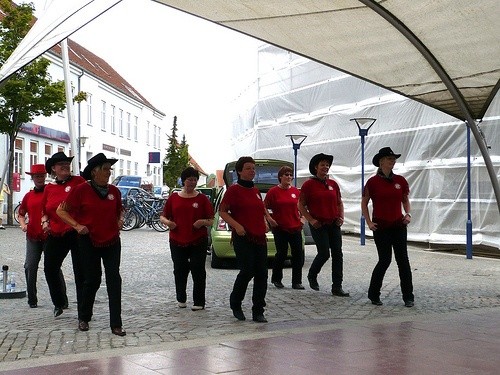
[368,295,382,305]
[293,283,304,289]
[309,278,320,290]
[273,281,284,288]
[230,303,246,321]
[404,301,414,307]
[178,302,187,308]
[191,305,203,311]
[30,303,38,308]
[253,313,266,323]
[331,289,349,297]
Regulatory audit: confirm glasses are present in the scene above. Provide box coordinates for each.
[283,173,293,177]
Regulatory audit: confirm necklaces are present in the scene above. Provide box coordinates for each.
[278,184,285,188]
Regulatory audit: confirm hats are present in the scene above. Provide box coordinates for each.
[309,153,333,175]
[372,147,402,167]
[25,164,48,175]
[45,152,74,176]
[83,153,118,179]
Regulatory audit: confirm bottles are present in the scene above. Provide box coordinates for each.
[0,269,15,292]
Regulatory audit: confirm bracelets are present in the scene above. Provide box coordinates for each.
[340,216,344,219]
[42,219,50,224]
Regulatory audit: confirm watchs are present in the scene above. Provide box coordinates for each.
[406,213,412,217]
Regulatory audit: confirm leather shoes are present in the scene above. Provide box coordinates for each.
[54,305,66,317]
[79,320,89,331]
[112,326,126,336]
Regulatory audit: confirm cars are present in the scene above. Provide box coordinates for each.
[210,159,306,269]
[108,175,219,232]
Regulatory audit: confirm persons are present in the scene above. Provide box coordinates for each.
[161,167,215,311]
[362,147,415,307]
[219,156,269,322]
[297,153,350,296]
[18,152,126,336]
[264,166,306,290]
[0,178,10,229]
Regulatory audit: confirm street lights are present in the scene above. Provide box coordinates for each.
[350,117,377,246]
[285,134,307,188]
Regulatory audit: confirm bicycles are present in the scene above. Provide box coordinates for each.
[14,201,29,224]
